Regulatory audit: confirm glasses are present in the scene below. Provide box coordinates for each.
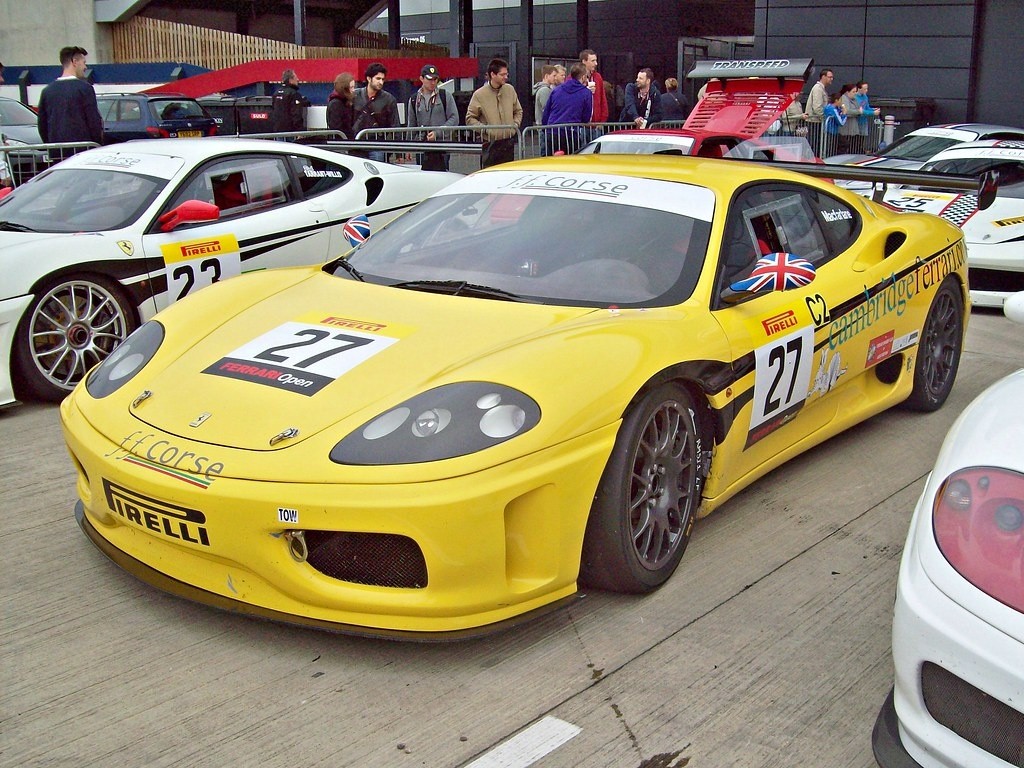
[826,75,834,79]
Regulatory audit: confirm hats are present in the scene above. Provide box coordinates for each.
[421,64,440,80]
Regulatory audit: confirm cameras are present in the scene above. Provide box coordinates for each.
[419,131,427,140]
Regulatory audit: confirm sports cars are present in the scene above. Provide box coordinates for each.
[0,138,493,411]
[55,150,971,645]
[823,121,1024,309]
[490,57,835,226]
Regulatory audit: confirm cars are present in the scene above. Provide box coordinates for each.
[871,290,1024,768]
[96,91,219,146]
[0,96,54,179]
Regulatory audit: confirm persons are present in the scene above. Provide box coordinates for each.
[269,69,306,142]
[783,69,881,159]
[325,73,361,156]
[353,63,404,164]
[465,58,524,169]
[531,49,688,157]
[38,45,103,166]
[405,64,460,171]
[697,77,719,101]
